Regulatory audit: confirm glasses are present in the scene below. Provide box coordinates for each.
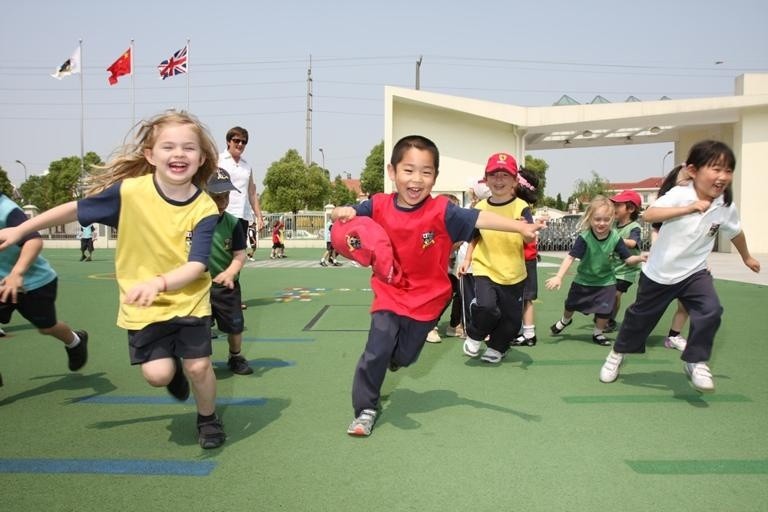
[231,139,247,144]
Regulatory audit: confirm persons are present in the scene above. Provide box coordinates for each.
[324,133,556,440]
[545,194,651,349]
[78,224,97,263]
[596,136,762,393]
[649,218,690,350]
[201,167,255,377]
[0,109,228,452]
[428,153,543,368]
[582,190,645,332]
[246,214,337,269]
[214,124,263,237]
[0,191,93,388]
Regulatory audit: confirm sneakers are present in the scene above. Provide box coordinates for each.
[64,328,88,371]
[425,318,714,393]
[347,408,378,435]
[196,414,224,450]
[168,357,189,400]
[228,356,252,375]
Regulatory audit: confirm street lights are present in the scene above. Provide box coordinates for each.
[17,159,28,182]
[660,150,673,182]
[319,148,325,179]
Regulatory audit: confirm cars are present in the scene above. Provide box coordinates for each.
[539,214,651,248]
[264,230,318,241]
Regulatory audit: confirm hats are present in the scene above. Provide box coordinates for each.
[206,167,241,193]
[609,191,641,208]
[486,154,517,176]
[473,183,492,199]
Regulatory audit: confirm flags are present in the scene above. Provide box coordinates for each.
[52,47,81,81]
[107,48,131,86]
[157,46,187,79]
